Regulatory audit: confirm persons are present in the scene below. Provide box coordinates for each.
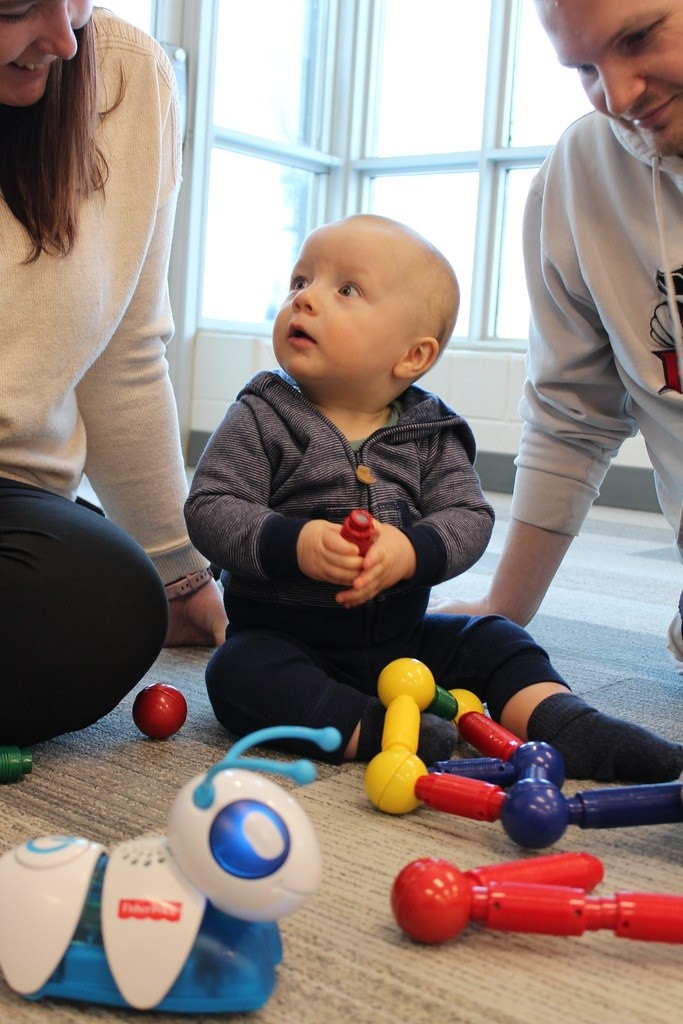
[430,0,683,655]
[1,0,230,745]
[182,214,682,786]
